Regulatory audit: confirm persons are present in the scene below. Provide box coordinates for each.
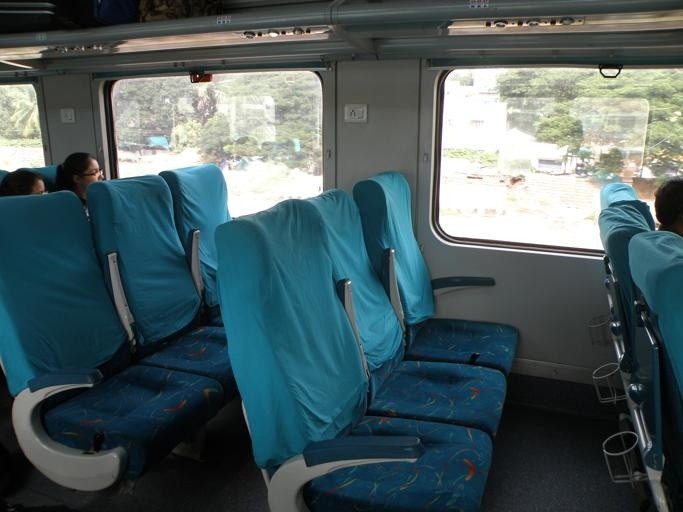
[655,178,683,237]
[54,152,104,222]
[0,171,48,196]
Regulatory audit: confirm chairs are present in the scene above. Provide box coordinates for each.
[352,170,519,369]
[213,200,493,510]
[596,183,683,510]
[1,165,242,493]
[306,189,507,434]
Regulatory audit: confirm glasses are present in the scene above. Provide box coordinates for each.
[77,168,105,177]
